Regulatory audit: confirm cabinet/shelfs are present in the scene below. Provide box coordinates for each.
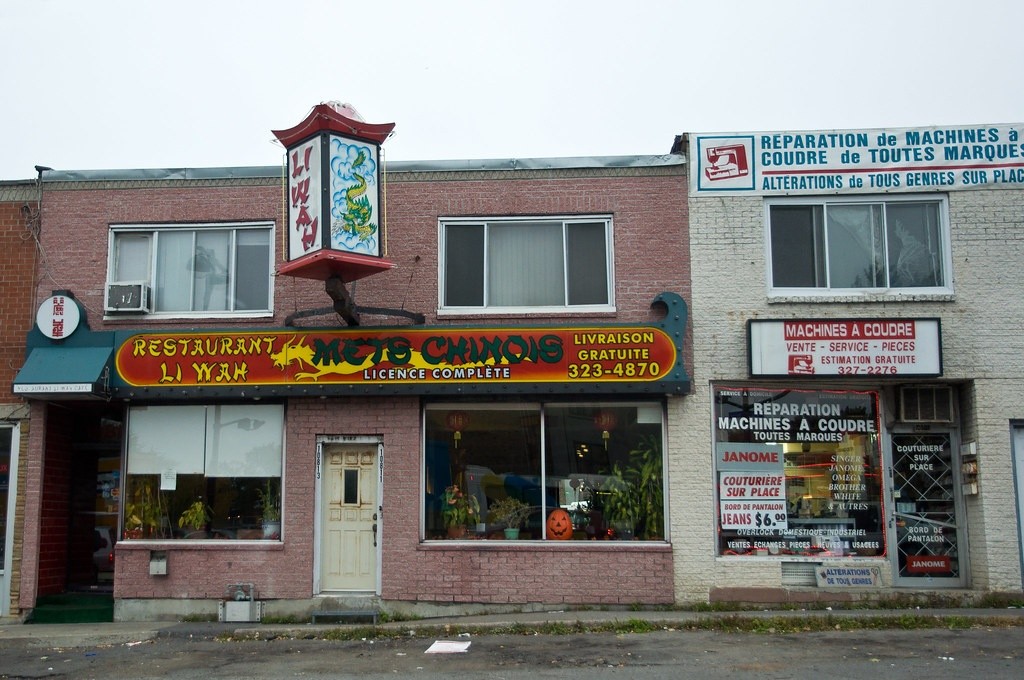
[719,518,857,555]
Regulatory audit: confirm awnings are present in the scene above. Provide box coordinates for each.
[12,345,114,395]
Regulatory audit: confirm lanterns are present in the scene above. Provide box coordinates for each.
[547,509,574,540]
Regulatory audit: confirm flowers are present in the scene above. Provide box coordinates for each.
[439,485,480,525]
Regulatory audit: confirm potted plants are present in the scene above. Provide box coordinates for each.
[255,480,281,540]
[122,477,167,539]
[177,502,210,538]
[488,496,533,540]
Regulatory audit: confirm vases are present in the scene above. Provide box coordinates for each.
[447,524,466,538]
